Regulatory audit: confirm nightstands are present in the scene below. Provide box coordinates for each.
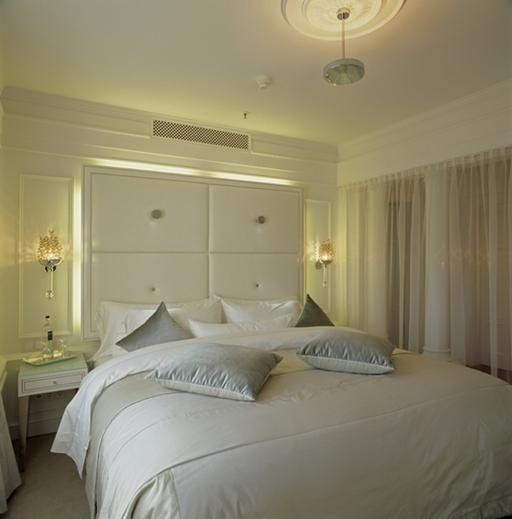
[17,353,89,473]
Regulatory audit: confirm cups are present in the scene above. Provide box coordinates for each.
[37,339,69,361]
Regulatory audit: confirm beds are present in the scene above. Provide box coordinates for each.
[87,349,511,518]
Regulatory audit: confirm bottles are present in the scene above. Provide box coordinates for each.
[40,315,55,358]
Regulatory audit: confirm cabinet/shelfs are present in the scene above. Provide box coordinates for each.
[73,165,306,344]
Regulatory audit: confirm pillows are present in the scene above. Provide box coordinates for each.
[214,296,302,331]
[292,294,335,326]
[296,329,395,375]
[116,302,192,354]
[189,313,296,338]
[144,343,283,401]
[94,298,222,358]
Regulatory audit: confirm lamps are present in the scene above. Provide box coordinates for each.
[282,1,405,87]
[318,240,334,288]
[36,224,63,296]
